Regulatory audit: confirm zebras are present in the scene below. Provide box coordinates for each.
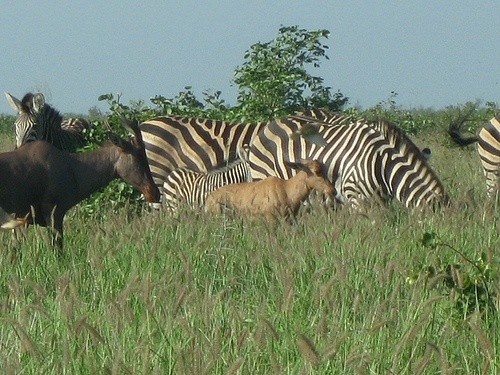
[138,108,452,218]
[443,98,500,206]
[5,91,90,153]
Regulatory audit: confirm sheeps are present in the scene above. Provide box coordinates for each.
[203,157,337,242]
[0,111,161,264]
